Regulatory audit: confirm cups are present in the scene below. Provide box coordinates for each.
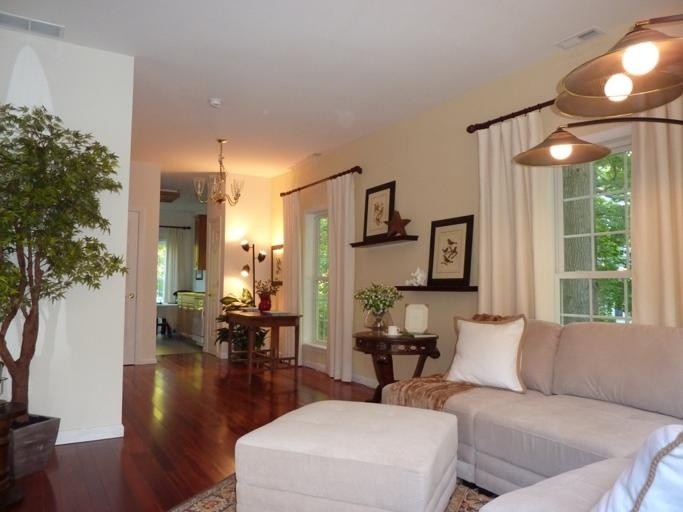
[387,325,400,335]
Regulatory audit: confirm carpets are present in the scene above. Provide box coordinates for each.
[168,469,497,512]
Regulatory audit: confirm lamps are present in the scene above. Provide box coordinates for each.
[193,135,243,210]
[558,12,683,100]
[239,239,267,305]
[552,74,682,118]
[508,115,683,171]
[160,188,178,204]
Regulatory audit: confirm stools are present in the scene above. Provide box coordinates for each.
[226,396,462,511]
[470,459,625,511]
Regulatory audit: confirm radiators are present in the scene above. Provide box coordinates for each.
[278,325,303,368]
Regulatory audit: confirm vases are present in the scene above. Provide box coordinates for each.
[257,292,271,313]
[363,310,392,330]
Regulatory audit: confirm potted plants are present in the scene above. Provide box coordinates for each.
[0,259,30,507]
[0,160,133,482]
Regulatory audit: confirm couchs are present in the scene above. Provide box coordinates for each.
[378,311,681,492]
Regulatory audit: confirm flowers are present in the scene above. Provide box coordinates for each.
[252,275,280,297]
[351,279,402,317]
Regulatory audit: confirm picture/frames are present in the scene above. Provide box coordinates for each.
[425,210,477,288]
[194,269,203,281]
[267,243,282,286]
[360,178,398,240]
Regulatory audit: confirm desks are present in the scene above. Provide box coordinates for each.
[224,308,303,387]
[350,330,440,407]
[155,303,183,339]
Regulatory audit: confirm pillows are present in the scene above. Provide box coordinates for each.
[589,421,681,512]
[443,315,528,397]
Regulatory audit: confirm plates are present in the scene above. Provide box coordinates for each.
[240,306,259,312]
[384,332,403,339]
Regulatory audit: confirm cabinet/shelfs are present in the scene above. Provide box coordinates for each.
[191,212,208,271]
